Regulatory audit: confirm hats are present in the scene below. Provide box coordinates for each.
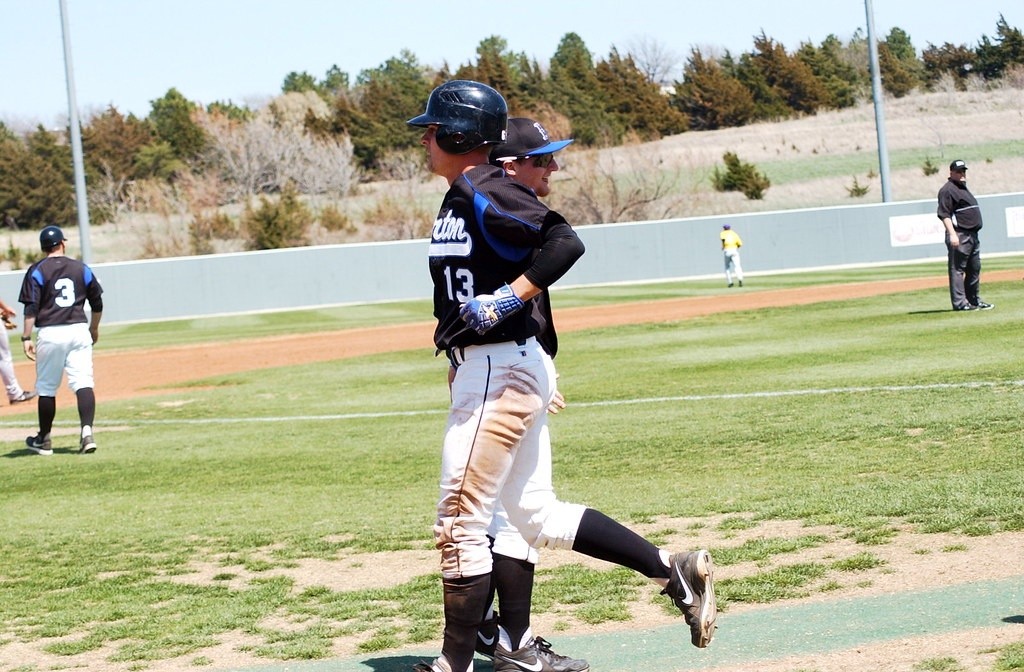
[949,160,969,173]
[484,118,573,166]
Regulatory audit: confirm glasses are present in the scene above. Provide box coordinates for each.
[516,154,555,174]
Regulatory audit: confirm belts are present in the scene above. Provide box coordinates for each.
[451,338,528,368]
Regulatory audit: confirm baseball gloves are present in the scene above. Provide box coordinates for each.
[0,311,17,329]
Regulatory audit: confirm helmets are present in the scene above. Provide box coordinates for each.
[38,226,69,247]
[407,79,509,153]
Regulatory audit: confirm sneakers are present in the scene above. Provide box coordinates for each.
[410,658,439,671]
[493,637,590,672]
[957,301,978,310]
[474,613,499,658]
[973,302,995,310]
[79,437,96,454]
[659,550,717,649]
[10,390,38,404]
[26,435,53,455]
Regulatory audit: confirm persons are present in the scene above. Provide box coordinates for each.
[937,160,995,311]
[0,299,37,404]
[18,226,104,456]
[720,224,743,288]
[404,79,717,672]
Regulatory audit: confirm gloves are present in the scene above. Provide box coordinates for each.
[457,283,523,336]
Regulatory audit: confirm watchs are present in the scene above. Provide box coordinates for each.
[21,336,31,343]
